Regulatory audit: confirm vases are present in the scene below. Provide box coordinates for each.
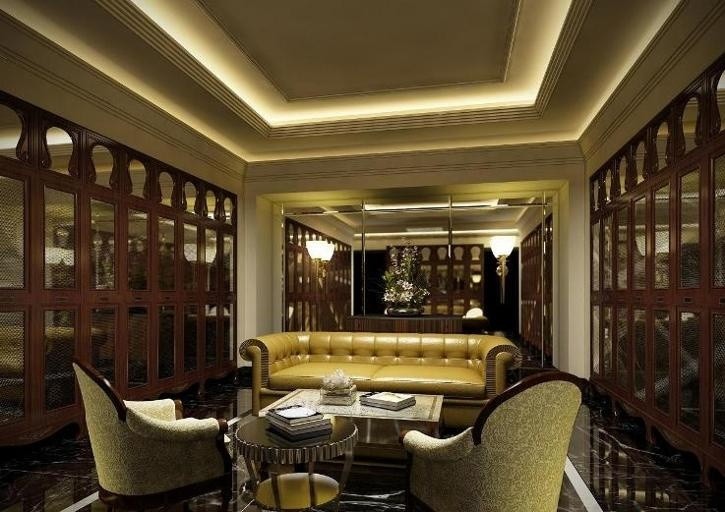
[383,306,422,317]
[319,385,358,406]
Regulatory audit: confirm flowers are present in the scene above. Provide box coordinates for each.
[381,237,432,314]
[321,367,353,390]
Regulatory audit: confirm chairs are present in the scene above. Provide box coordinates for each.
[68,354,231,506]
[398,371,585,510]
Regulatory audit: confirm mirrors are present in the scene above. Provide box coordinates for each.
[281,202,546,373]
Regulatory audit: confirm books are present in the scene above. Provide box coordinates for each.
[360,399,416,411]
[265,404,334,443]
[358,391,416,407]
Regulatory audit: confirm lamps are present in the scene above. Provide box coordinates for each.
[304,240,334,281]
[490,235,515,304]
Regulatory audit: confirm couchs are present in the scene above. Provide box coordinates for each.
[239,331,522,428]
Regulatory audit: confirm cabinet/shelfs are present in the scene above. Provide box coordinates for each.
[416,262,484,317]
[285,248,352,332]
